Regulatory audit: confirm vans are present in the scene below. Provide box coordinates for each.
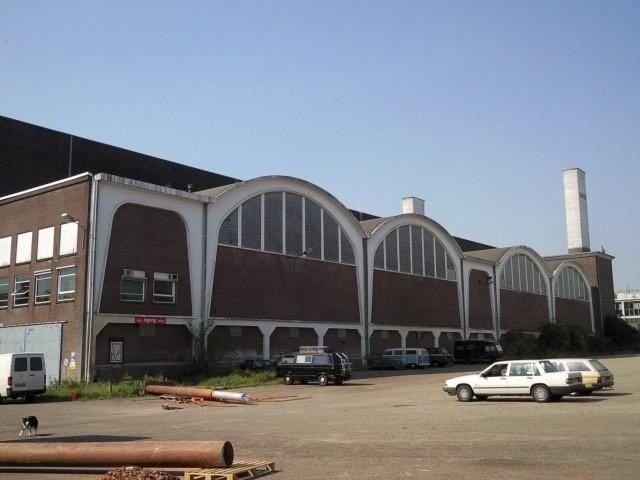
[384,348,431,369]
[276,351,352,386]
[0,353,46,402]
[454,340,504,361]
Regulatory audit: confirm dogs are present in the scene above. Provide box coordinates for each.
[18,415,38,438]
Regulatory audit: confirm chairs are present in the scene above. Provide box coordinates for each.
[518,366,528,376]
[510,367,518,376]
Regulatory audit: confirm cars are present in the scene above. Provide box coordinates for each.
[427,347,451,367]
[575,359,614,395]
[443,360,585,402]
